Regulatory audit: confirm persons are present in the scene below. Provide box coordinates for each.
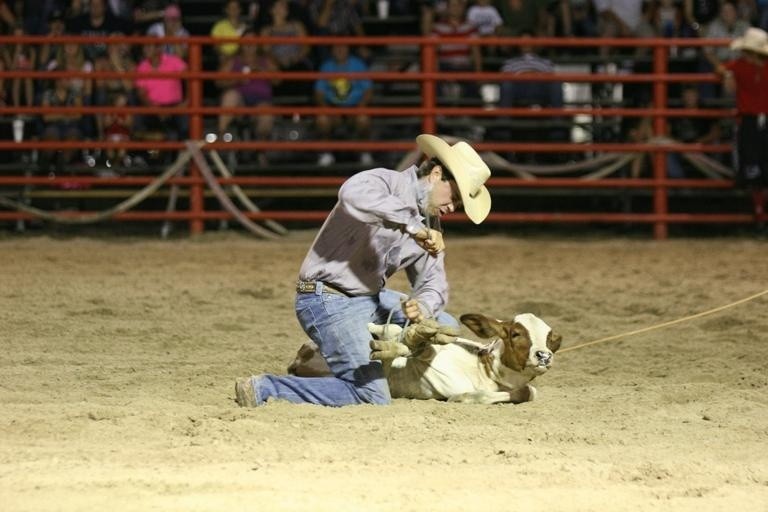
[234,133,492,408]
[433,0,768,244]
[2,1,372,168]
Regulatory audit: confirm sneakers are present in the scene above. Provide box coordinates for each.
[235,375,257,408]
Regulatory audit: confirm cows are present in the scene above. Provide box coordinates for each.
[286,312,564,405]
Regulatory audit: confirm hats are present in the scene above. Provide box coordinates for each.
[417,133,493,226]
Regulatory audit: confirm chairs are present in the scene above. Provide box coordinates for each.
[0,2,768,198]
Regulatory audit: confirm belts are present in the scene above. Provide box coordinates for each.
[294,279,348,296]
